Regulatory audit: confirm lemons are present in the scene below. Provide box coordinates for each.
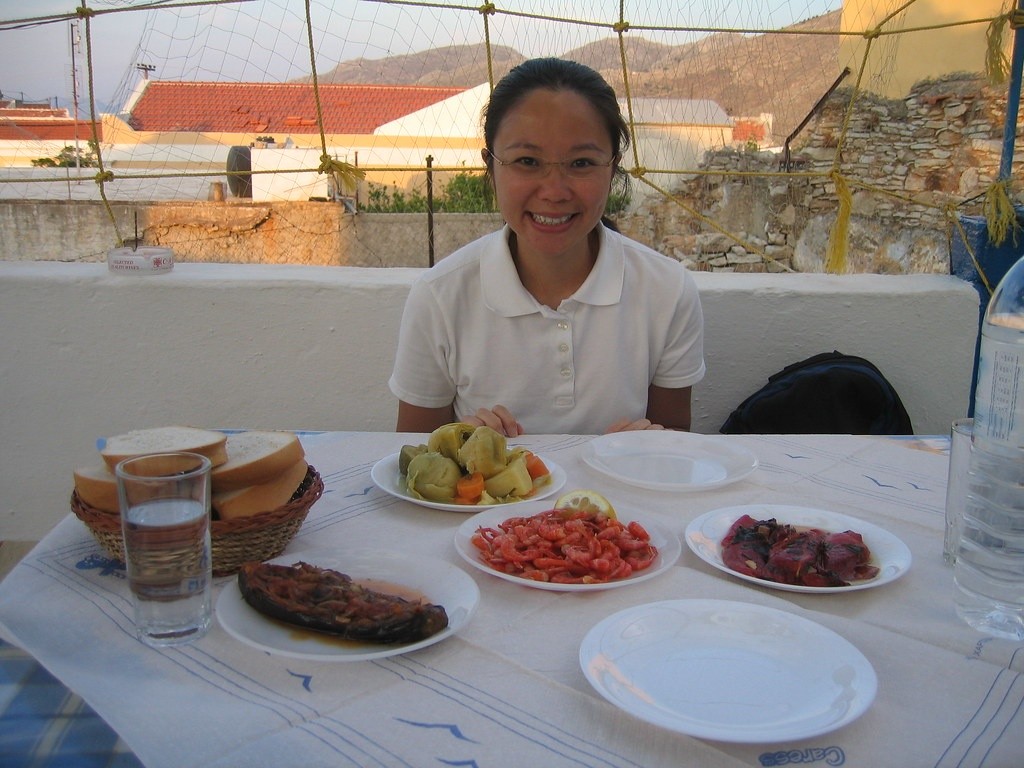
[554,489,617,519]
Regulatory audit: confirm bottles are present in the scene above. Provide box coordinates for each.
[953,256,1024,639]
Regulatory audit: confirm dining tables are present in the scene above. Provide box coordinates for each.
[1,429,1024,768]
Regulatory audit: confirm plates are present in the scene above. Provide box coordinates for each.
[583,430,760,491]
[217,544,479,661]
[578,599,878,741]
[371,446,567,511]
[455,501,683,591]
[685,503,913,592]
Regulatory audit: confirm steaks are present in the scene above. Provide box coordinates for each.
[236,562,448,644]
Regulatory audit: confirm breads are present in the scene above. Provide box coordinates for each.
[72,424,309,521]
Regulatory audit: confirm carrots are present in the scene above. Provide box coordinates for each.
[457,472,486,499]
[524,453,550,479]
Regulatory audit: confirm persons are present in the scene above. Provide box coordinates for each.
[387,57,706,435]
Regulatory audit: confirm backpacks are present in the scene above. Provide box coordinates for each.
[718,349,916,436]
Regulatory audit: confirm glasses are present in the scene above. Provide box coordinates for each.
[485,146,616,180]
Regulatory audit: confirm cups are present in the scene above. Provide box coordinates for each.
[116,451,211,646]
[944,419,974,567]
